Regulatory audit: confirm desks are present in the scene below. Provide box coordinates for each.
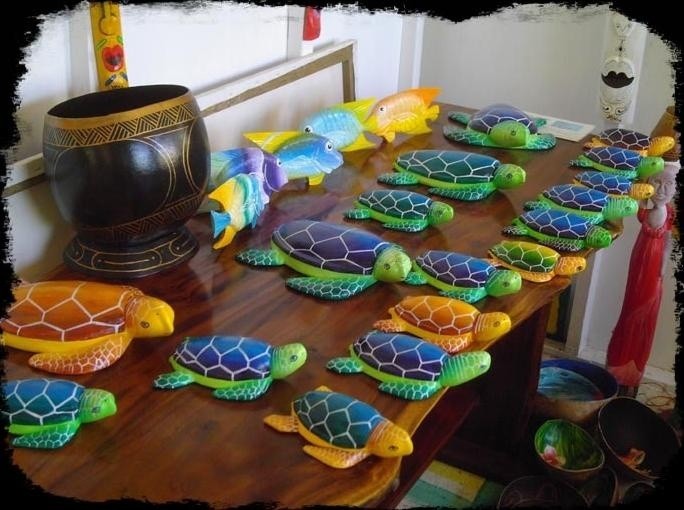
[1,99,597,510]
